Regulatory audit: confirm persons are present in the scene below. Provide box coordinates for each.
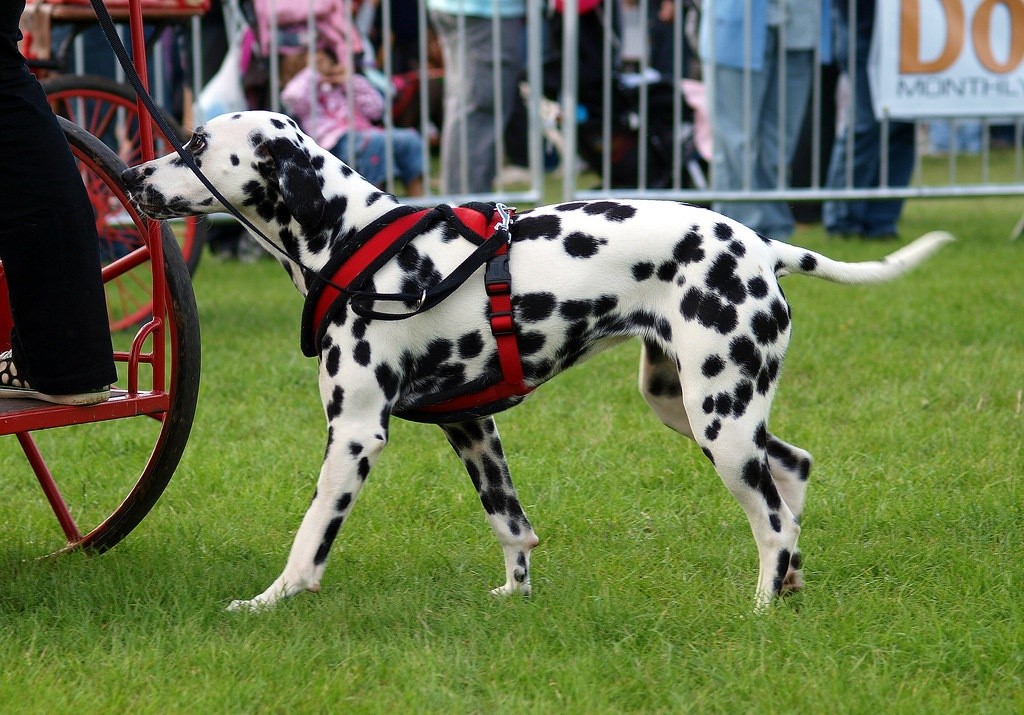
[278,1,920,243]
[0,0,121,405]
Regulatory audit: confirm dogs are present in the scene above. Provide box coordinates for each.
[120,109,954,613]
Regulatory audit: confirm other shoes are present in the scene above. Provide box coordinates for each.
[0,348,110,405]
